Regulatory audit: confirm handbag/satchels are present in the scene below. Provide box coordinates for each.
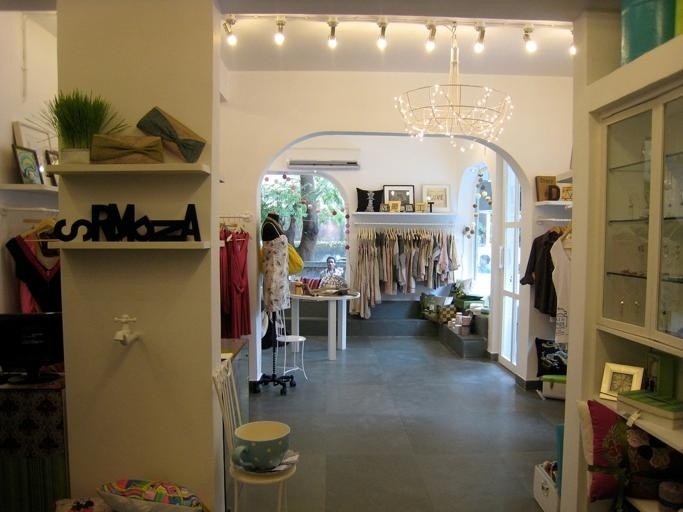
[258,217,304,275]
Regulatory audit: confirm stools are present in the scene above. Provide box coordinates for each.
[541,374,566,400]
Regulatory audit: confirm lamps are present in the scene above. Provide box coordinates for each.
[474,25,485,54]
[521,32,537,53]
[374,20,391,52]
[326,19,340,48]
[222,20,238,47]
[427,25,436,53]
[271,19,287,45]
[396,26,515,152]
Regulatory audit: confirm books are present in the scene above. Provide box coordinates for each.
[534,176,556,201]
[618,390,683,430]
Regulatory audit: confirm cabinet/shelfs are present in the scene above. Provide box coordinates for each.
[595,87,683,350]
[45,162,211,250]
[594,328,683,512]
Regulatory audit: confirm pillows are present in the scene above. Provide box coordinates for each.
[355,187,383,212]
[535,337,568,377]
[95,479,209,512]
[574,400,682,502]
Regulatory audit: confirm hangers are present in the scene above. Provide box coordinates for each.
[356,222,454,241]
[21,208,56,242]
[220,214,252,242]
[547,217,571,242]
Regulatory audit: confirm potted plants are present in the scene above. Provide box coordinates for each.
[21,84,133,164]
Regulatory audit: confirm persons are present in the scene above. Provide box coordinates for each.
[478,255,491,274]
[319,257,341,281]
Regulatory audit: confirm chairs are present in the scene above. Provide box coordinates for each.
[212,356,298,512]
[275,310,310,386]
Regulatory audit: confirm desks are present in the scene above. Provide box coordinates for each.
[290,291,361,361]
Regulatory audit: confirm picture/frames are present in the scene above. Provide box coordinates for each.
[600,362,645,401]
[383,185,415,212]
[423,184,450,213]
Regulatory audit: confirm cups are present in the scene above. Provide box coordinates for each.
[231,420,291,473]
